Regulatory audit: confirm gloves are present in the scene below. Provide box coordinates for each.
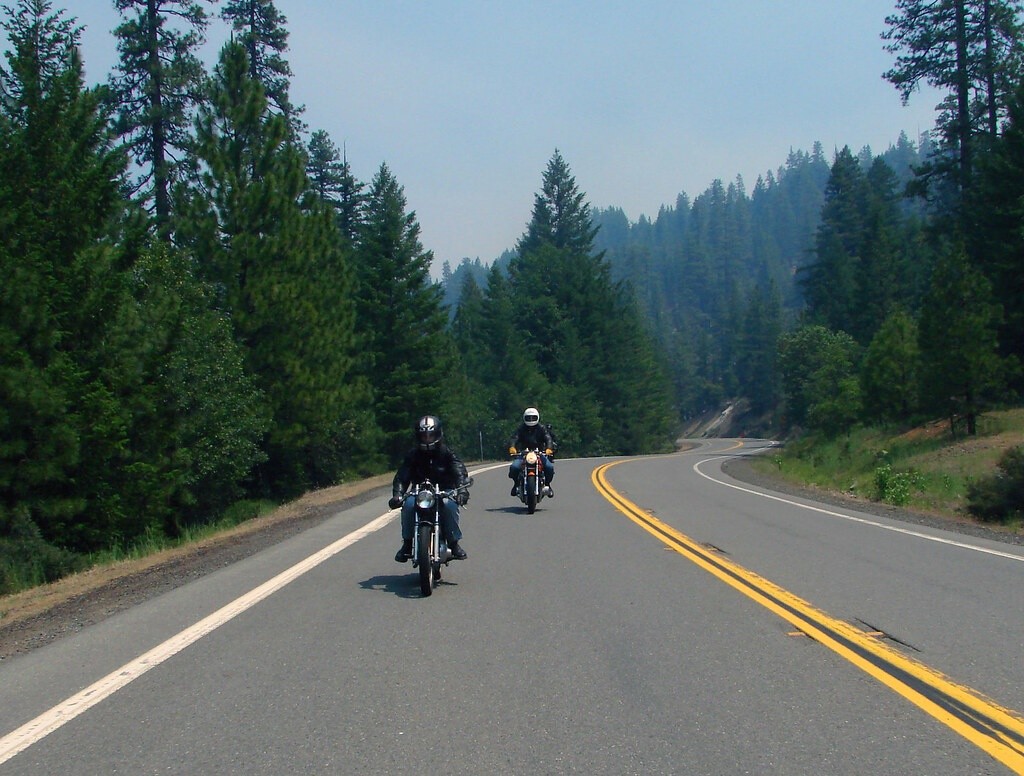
[510,447,516,454]
[457,488,469,505]
[389,496,402,509]
[546,449,552,455]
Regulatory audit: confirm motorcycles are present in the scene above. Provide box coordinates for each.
[396,475,474,594]
[510,446,554,515]
[549,441,560,464]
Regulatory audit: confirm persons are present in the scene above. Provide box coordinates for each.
[509,408,555,498]
[389,417,469,563]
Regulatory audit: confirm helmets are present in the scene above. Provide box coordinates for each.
[415,414,443,449]
[523,408,540,426]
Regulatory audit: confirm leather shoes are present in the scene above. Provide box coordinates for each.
[395,543,412,562]
[447,543,467,559]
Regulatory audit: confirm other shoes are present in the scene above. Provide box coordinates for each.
[511,485,517,496]
[548,489,554,497]
